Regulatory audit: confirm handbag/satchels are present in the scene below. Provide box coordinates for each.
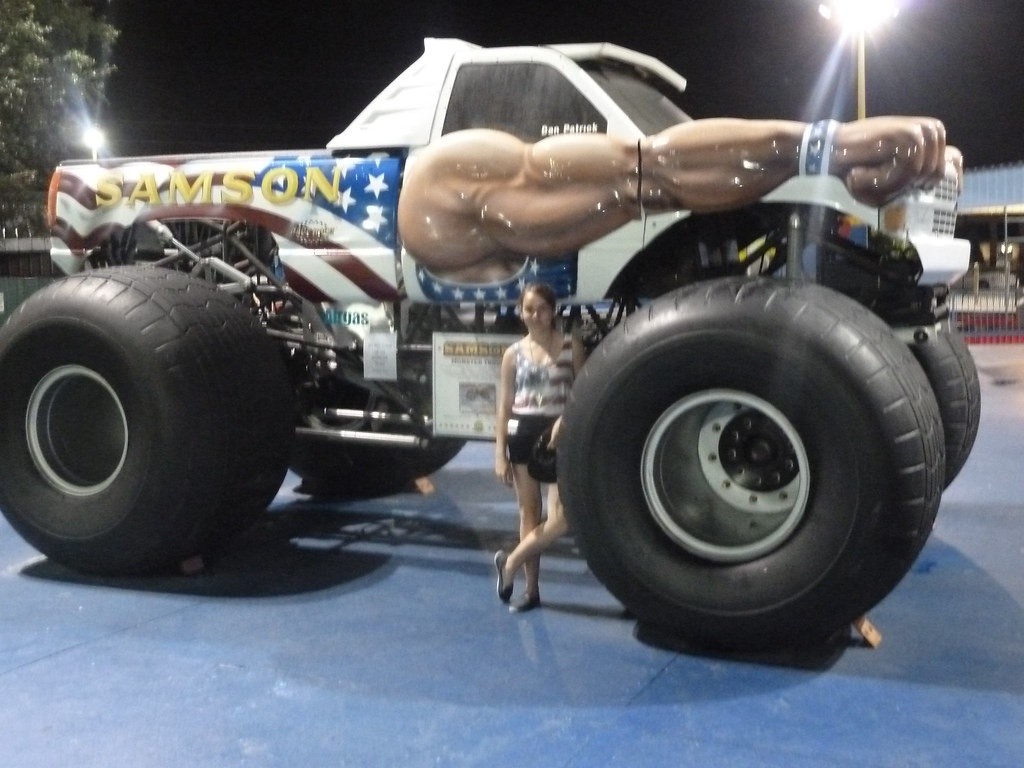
[527,423,557,483]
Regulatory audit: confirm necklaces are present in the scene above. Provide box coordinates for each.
[527,336,549,366]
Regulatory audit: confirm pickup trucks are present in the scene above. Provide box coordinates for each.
[1,31,982,647]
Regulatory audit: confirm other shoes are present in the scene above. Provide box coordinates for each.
[509,594,540,613]
[494,550,513,601]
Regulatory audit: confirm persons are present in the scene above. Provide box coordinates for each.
[396,115,946,266]
[493,282,585,613]
